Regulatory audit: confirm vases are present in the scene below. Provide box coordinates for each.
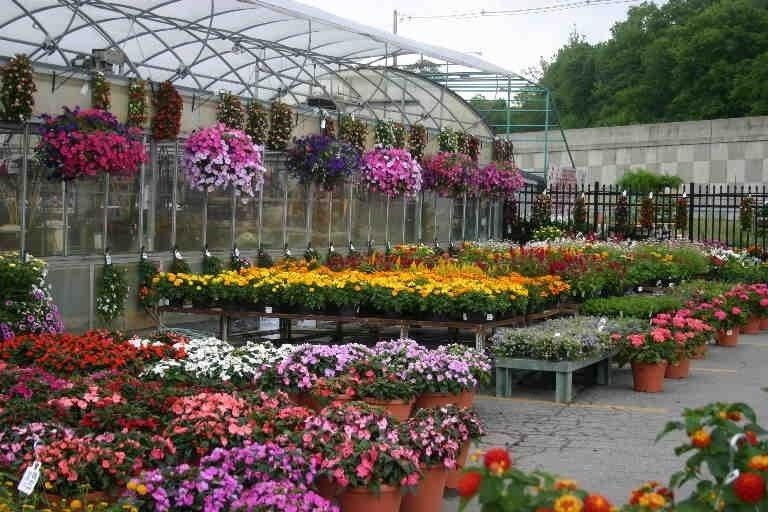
[629,350,689,391]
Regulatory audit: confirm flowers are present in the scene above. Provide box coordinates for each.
[454,402,765,512]
[1,251,492,511]
[152,191,768,371]
[1,51,526,207]
